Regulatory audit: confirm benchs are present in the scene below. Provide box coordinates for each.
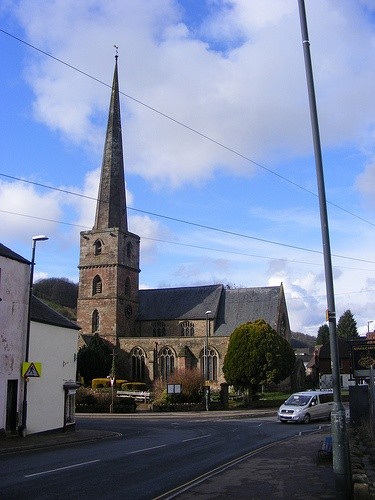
[317,436,334,463]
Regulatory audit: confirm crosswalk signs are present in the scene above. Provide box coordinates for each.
[21,361,42,380]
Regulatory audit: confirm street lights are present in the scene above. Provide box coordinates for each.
[205,310,212,380]
[20,235,50,434]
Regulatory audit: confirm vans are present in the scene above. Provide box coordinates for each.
[276,391,334,423]
